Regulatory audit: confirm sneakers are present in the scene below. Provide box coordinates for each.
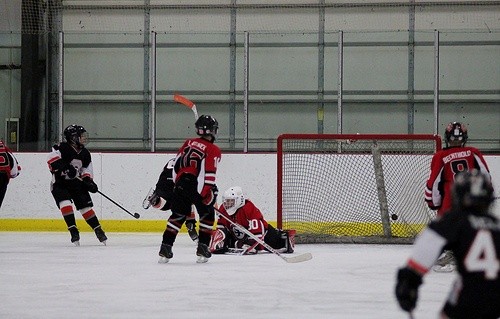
[432,251,457,273]
[187,228,199,245]
[143,186,160,210]
[158,243,173,264]
[94,225,108,246]
[68,225,80,246]
[196,242,212,264]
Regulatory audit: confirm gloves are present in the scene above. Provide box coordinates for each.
[201,183,218,206]
[83,177,98,193]
[395,268,423,312]
[425,200,442,211]
[239,243,257,255]
[211,245,230,254]
[63,163,76,178]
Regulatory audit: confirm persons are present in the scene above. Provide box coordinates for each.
[424,121,492,271]
[158,114,222,262]
[396,170,500,319]
[0,140,21,208]
[47,124,108,245]
[210,186,296,255]
[149,156,198,244]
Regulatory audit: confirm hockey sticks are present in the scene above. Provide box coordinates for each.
[409,311,414,319]
[175,96,199,121]
[97,190,140,218]
[391,214,398,220]
[214,207,312,263]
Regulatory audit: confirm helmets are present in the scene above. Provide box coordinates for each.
[64,124,87,148]
[221,187,245,216]
[443,122,470,149]
[195,114,220,143]
[451,169,492,213]
